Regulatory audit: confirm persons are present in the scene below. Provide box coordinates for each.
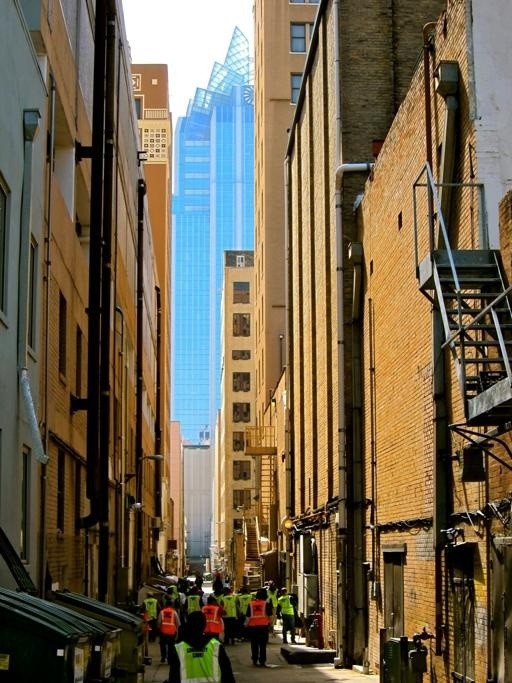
[141,568,298,667]
[167,611,236,683]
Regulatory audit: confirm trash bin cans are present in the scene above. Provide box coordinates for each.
[0,588,152,683]
